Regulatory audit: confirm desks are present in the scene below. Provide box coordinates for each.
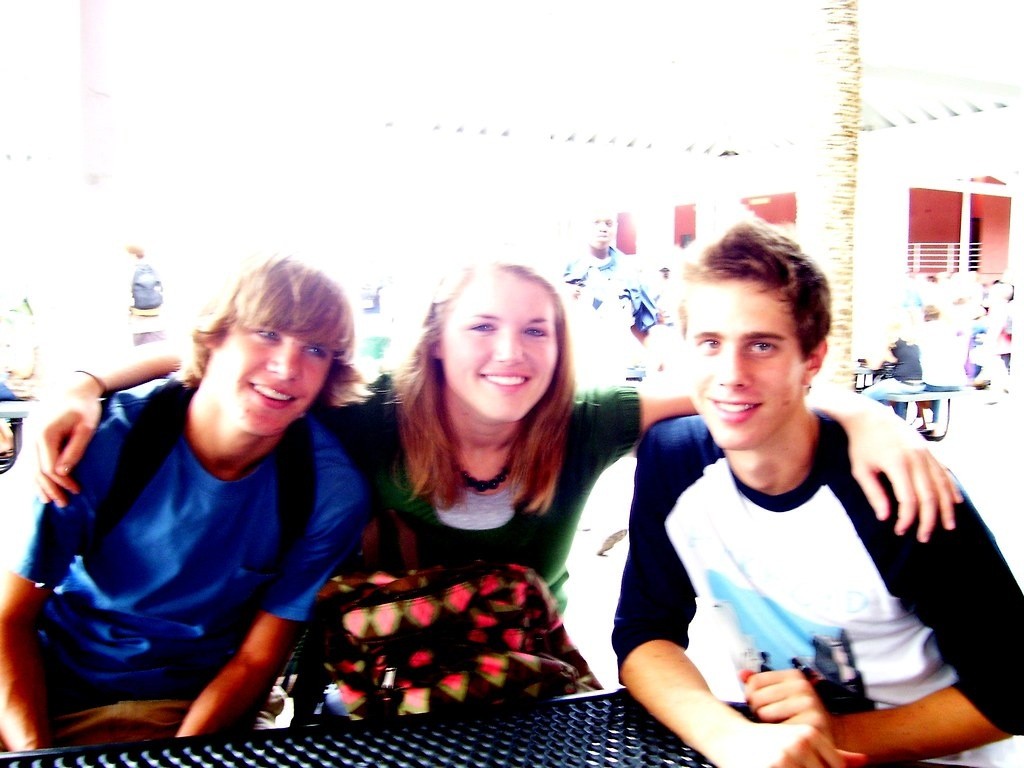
[0,688,970,768]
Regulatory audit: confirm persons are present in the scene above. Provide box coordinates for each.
[122,246,165,346]
[862,267,1016,423]
[550,213,683,402]
[35,256,966,730]
[0,246,370,754]
[613,224,1024,768]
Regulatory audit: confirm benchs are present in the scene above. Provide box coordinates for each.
[886,390,978,439]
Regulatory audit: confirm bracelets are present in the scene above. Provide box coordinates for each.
[76,370,106,392]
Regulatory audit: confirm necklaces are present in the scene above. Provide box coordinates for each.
[451,463,509,492]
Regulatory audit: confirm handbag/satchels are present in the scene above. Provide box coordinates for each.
[305,560,602,724]
